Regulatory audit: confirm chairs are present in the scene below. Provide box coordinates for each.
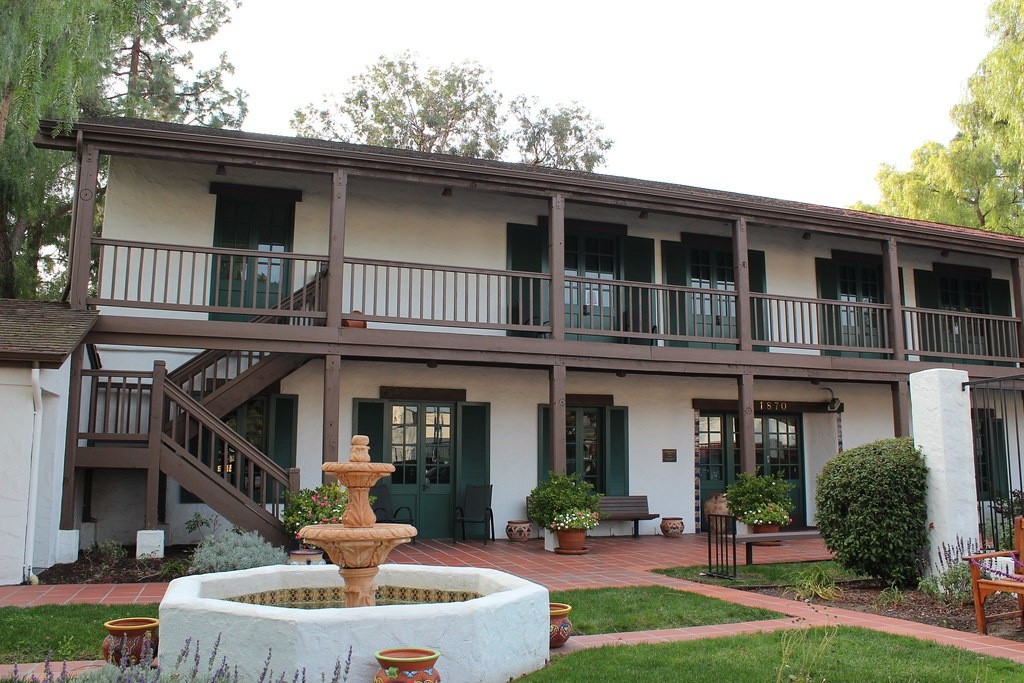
[369,486,417,546]
[623,310,658,347]
[452,483,496,546]
[516,310,550,339]
[963,514,1024,635]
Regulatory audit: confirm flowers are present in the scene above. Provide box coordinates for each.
[281,485,380,542]
[723,465,796,528]
[528,470,612,534]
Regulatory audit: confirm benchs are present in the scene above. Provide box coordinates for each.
[526,495,660,539]
[718,530,821,564]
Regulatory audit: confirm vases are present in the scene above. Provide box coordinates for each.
[342,311,367,329]
[505,520,532,541]
[101,616,159,666]
[374,648,441,683]
[660,518,685,537]
[549,603,572,649]
[555,529,587,550]
[753,526,781,547]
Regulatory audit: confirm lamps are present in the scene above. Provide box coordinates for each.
[638,211,648,220]
[820,387,841,411]
[801,232,812,240]
[940,250,949,257]
[442,185,453,197]
[214,166,226,176]
